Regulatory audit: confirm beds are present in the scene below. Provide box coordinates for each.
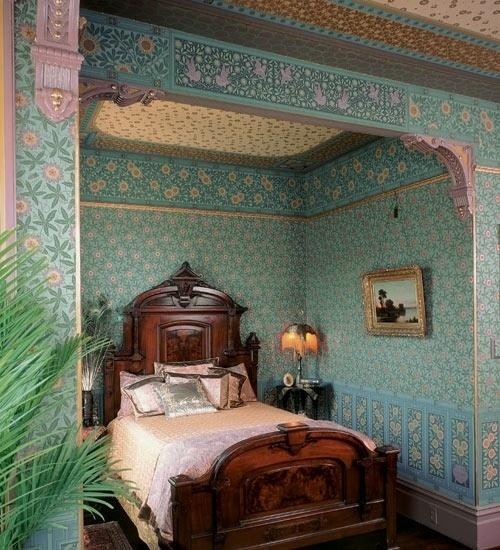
[97,259,405,549]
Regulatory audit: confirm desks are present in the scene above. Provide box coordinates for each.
[276,378,334,423]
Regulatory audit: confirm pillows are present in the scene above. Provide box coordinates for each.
[114,356,259,425]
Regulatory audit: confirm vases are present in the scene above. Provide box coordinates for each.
[80,390,96,427]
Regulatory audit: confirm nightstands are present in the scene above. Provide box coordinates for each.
[79,423,118,525]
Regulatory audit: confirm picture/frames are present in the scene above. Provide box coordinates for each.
[283,373,294,387]
[357,262,428,340]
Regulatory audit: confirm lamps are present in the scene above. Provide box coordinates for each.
[279,321,322,387]
